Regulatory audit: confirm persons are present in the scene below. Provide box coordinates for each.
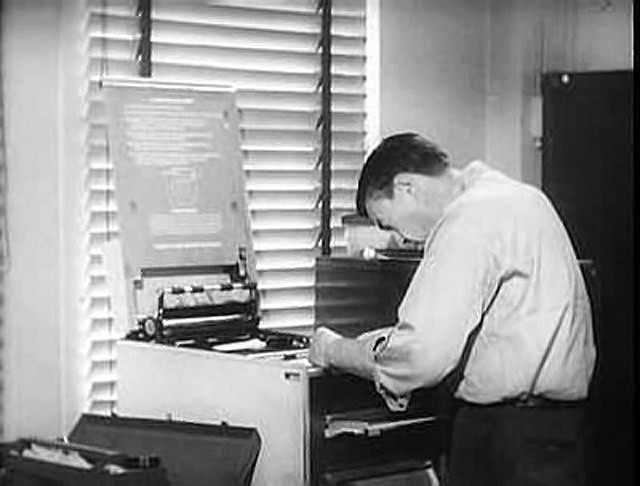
[306,131,599,486]
